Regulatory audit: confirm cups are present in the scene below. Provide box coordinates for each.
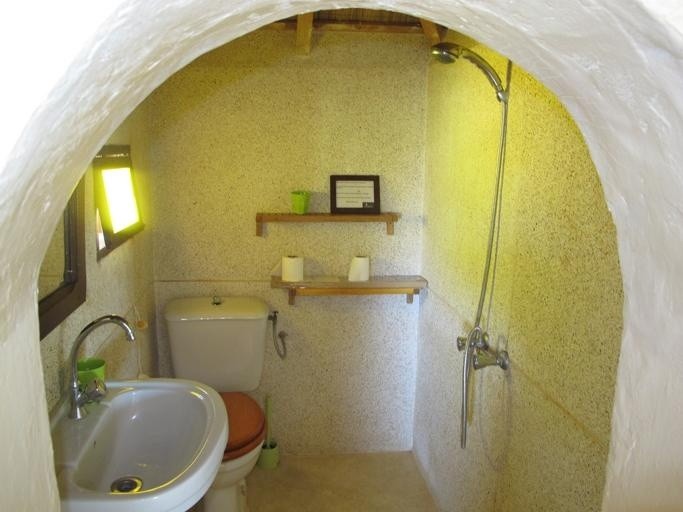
[291,190,311,214]
[76,358,106,402]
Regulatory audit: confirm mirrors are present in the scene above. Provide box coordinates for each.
[37,175,86,341]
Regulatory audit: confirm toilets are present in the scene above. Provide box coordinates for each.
[162,295,270,512]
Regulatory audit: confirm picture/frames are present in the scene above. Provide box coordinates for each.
[329,174,380,215]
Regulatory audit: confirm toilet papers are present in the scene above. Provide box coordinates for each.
[281,255,304,283]
[348,256,370,282]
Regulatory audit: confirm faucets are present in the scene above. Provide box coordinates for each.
[68,313,136,421]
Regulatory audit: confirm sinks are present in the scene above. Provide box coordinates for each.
[48,377,230,512]
